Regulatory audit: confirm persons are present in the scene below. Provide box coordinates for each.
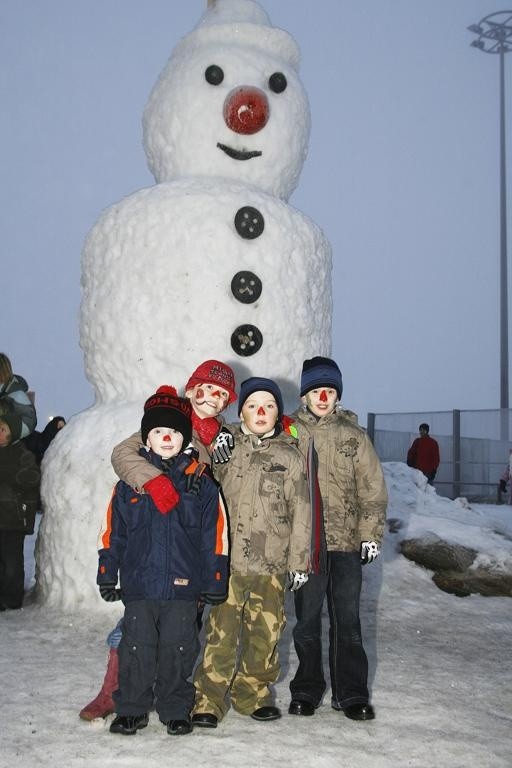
[207,357,387,721]
[0,354,65,612]
[407,423,440,487]
[95,385,228,736]
[191,377,312,728]
[81,360,238,722]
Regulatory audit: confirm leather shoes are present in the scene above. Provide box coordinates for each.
[289,700,314,715]
[252,706,280,720]
[192,713,217,727]
[332,702,374,720]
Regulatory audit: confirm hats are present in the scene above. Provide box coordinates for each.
[300,357,342,399]
[238,377,284,421]
[421,424,429,432]
[141,385,191,451]
[1,413,22,443]
[184,360,238,404]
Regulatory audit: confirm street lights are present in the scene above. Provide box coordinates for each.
[467,9,511,412]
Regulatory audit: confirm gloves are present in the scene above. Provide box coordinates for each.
[361,541,380,565]
[500,480,507,493]
[200,594,228,606]
[211,426,234,464]
[144,476,178,514]
[99,585,129,601]
[286,572,308,591]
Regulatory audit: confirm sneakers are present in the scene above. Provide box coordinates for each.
[110,713,149,734]
[161,720,192,735]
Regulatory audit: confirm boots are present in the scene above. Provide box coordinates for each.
[79,647,119,720]
